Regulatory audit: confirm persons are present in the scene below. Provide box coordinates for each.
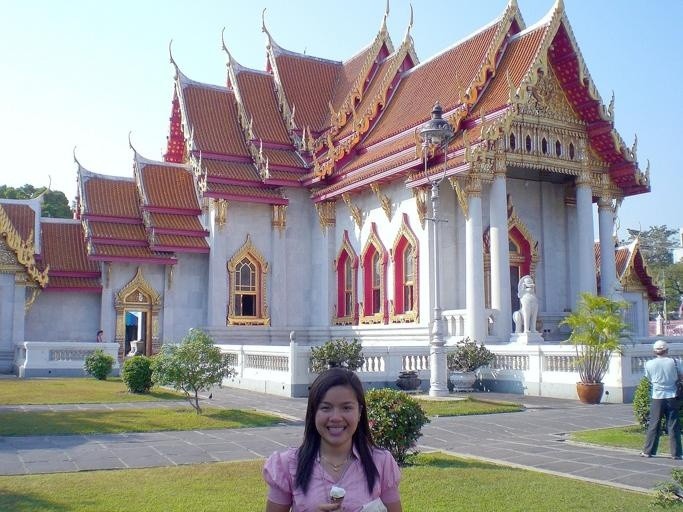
[639,339,682,459]
[262,369,401,512]
[96,330,103,342]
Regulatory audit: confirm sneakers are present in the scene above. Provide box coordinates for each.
[673,455,683,460]
[640,452,650,457]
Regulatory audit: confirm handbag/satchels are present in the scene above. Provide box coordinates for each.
[676,379,683,401]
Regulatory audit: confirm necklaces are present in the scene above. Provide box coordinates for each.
[321,448,344,472]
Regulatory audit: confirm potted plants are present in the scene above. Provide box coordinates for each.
[557,292,636,404]
[446,337,494,391]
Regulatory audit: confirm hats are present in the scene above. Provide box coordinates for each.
[654,340,668,351]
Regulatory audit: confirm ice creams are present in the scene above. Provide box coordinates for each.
[330,486,345,511]
